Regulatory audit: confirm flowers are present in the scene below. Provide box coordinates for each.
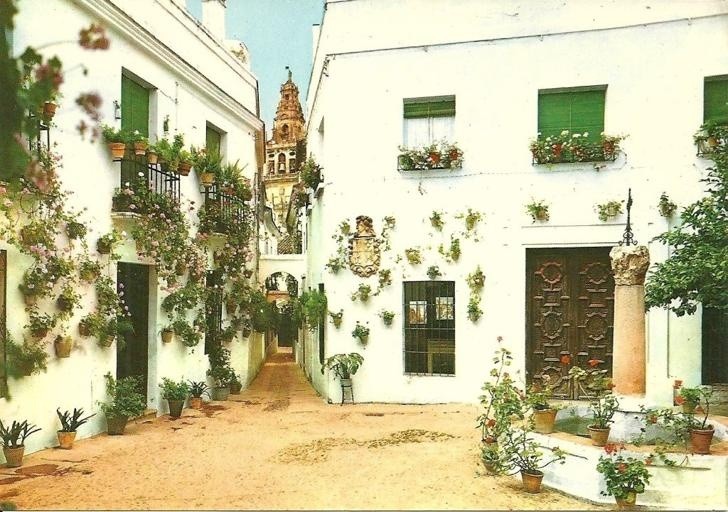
[479,336,528,458]
[527,375,559,408]
[173,288,229,348]
[97,226,128,243]
[543,134,567,150]
[18,268,54,294]
[0,136,65,268]
[561,128,592,148]
[527,131,549,149]
[599,130,630,143]
[108,170,186,221]
[596,455,653,497]
[132,220,211,286]
[199,195,256,287]
[442,135,463,169]
[560,355,619,428]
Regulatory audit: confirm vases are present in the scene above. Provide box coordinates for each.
[585,423,612,448]
[23,292,38,308]
[528,147,544,158]
[571,145,584,157]
[550,143,563,155]
[96,238,113,256]
[447,147,457,161]
[481,455,503,478]
[603,140,615,152]
[609,488,637,510]
[530,403,559,435]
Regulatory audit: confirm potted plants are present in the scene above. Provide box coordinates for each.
[379,311,396,326]
[319,352,364,386]
[465,208,481,230]
[56,406,97,449]
[157,375,186,418]
[156,141,173,173]
[359,283,371,302]
[243,322,252,338]
[130,129,150,155]
[65,205,87,239]
[638,386,713,469]
[95,370,146,437]
[24,310,59,339]
[102,124,132,160]
[700,116,728,148]
[497,425,568,492]
[412,146,428,164]
[466,295,483,322]
[674,387,701,415]
[42,91,60,118]
[526,199,550,224]
[352,319,368,346]
[200,157,215,188]
[101,316,134,350]
[328,309,345,327]
[187,380,212,410]
[58,279,82,317]
[78,311,102,337]
[597,201,621,223]
[179,151,194,177]
[144,144,158,166]
[230,370,243,395]
[55,325,72,359]
[397,144,411,166]
[0,419,42,468]
[159,324,172,343]
[428,139,442,166]
[212,370,230,402]
